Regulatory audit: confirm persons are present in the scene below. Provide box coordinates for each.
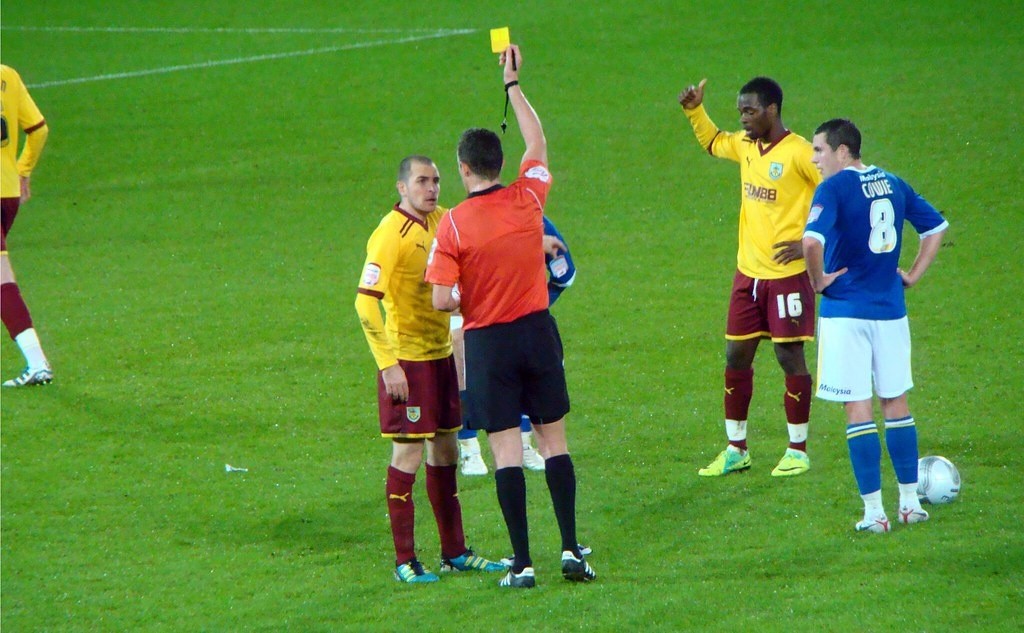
[803,119,950,531]
[0,63,53,387]
[355,156,568,583]
[451,217,577,475]
[678,77,825,477]
[425,45,593,588]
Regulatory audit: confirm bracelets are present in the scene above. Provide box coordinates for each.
[505,81,518,89]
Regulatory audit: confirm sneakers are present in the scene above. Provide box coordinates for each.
[460,444,489,476]
[855,513,891,535]
[771,452,810,476]
[440,547,506,574]
[698,446,751,477]
[3,361,54,386]
[898,504,929,524]
[497,555,535,588]
[560,543,596,581]
[524,445,545,471]
[394,555,439,583]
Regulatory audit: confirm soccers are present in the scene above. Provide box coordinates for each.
[915,455,961,505]
